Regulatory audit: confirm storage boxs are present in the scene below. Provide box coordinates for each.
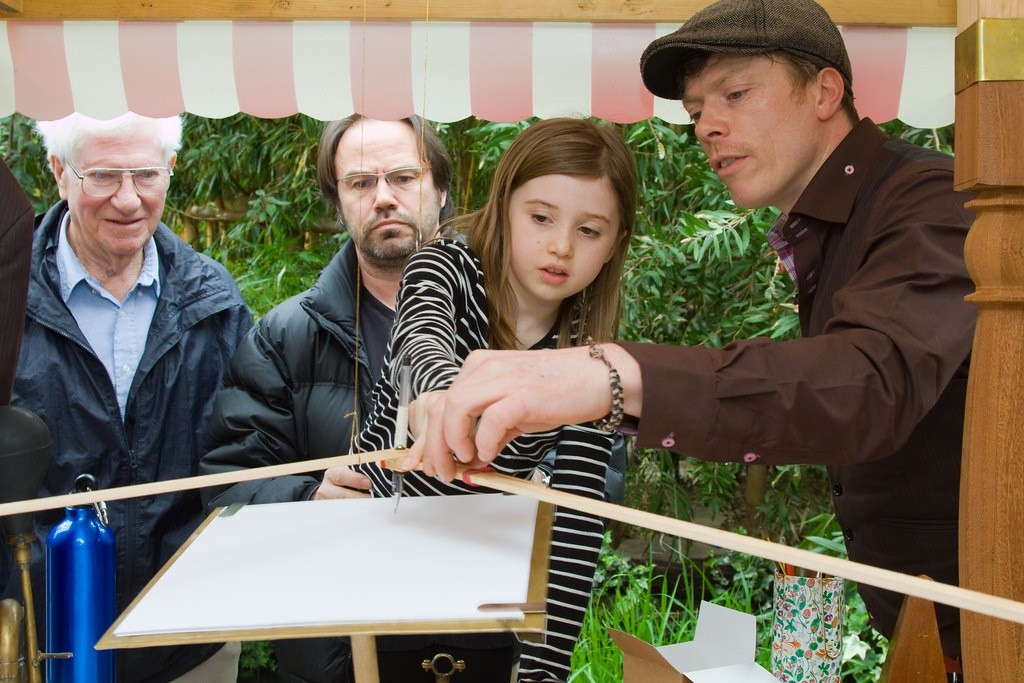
[605,600,787,683]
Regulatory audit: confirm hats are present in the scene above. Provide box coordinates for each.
[640,0,853,100]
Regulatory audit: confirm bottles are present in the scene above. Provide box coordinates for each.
[44,472,117,683]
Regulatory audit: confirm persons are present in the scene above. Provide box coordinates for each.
[442,2,981,683]
[0,112,253,683]
[198,113,637,683]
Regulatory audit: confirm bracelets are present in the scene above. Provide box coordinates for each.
[586,335,625,433]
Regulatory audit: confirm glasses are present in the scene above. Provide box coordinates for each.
[337,166,422,191]
[66,161,174,198]
